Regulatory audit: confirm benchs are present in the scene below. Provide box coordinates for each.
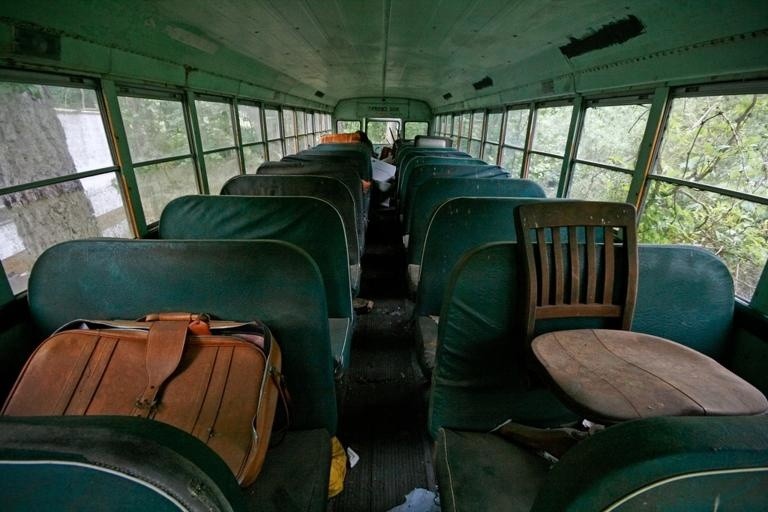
[2,408,254,511]
[530,405,768,512]
[393,137,512,286]
[427,240,739,512]
[214,135,376,297]
[157,192,359,388]
[25,235,338,510]
[411,193,607,380]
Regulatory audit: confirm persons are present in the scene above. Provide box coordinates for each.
[356,131,421,224]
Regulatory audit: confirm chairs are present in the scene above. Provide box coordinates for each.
[509,198,768,485]
